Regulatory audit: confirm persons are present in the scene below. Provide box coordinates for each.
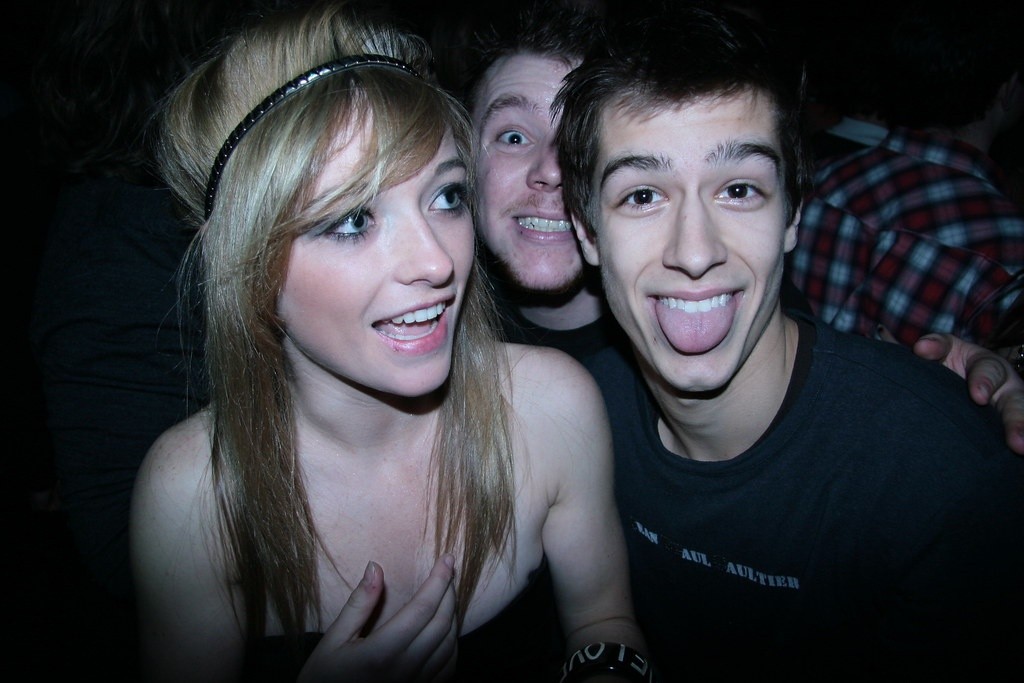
[29,3,649,683]
[469,1,1024,683]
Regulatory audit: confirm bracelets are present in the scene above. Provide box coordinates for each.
[559,641,652,683]
